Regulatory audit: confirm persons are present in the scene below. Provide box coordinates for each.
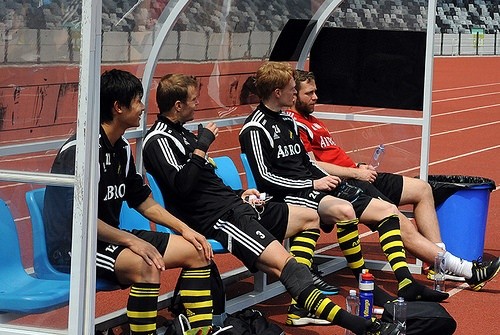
[142,74,406,334]
[283,69,500,291]
[43,69,213,335]
[238,61,449,307]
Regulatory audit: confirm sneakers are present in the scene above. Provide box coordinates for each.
[361,289,398,305]
[398,283,449,303]
[354,317,404,335]
[306,265,340,295]
[465,257,499,292]
[426,264,468,283]
[284,303,334,327]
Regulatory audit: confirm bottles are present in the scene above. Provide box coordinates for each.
[434,251,446,293]
[359,269,375,319]
[344,290,359,335]
[393,297,407,335]
[367,144,385,171]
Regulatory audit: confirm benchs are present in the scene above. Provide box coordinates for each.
[0,153,261,315]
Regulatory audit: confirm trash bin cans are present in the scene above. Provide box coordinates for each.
[413,175,496,265]
